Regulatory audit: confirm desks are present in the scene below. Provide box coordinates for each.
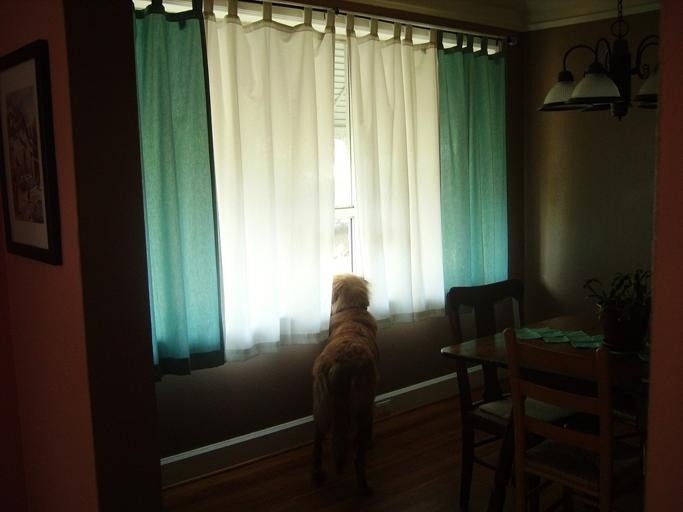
[440,313,649,512]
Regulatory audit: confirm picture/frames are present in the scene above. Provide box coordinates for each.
[0,40,63,266]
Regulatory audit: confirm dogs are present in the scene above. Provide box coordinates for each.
[312,273,378,498]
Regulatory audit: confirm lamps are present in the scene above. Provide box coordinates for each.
[536,0,660,119]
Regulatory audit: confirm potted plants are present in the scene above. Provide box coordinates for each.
[583,268,651,351]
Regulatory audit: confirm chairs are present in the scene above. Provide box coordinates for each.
[445,279,543,512]
[503,326,648,512]
